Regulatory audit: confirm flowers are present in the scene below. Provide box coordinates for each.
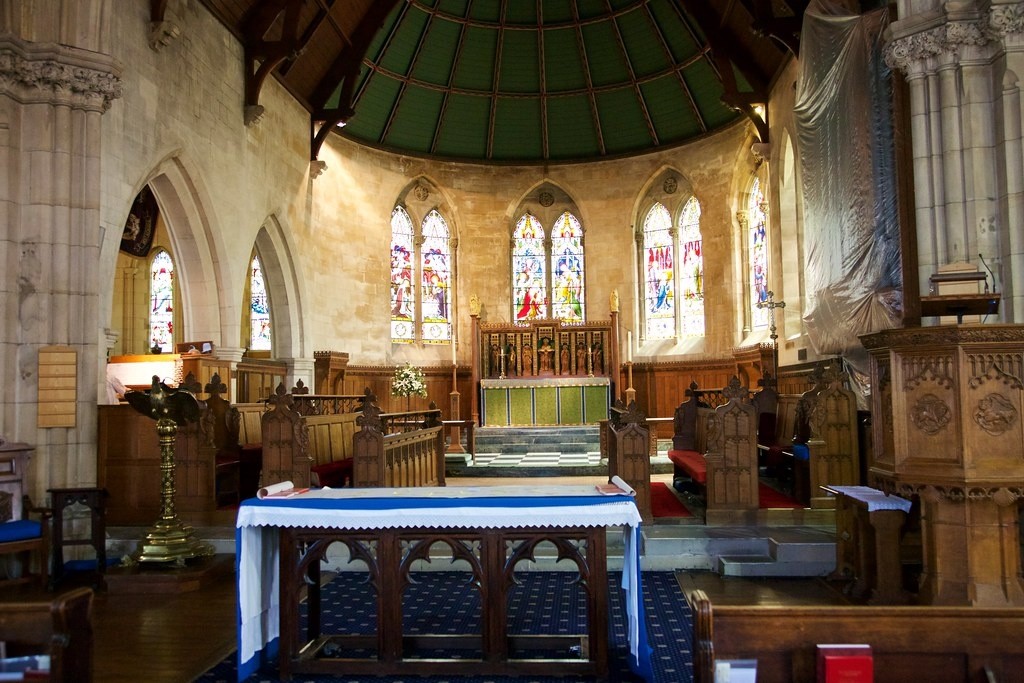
[390,360,428,400]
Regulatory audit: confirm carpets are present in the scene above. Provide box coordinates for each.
[758,482,808,508]
[189,566,697,683]
[649,482,694,517]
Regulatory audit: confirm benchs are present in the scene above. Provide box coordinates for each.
[97,388,445,525]
[605,369,858,526]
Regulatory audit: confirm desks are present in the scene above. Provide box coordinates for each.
[240,484,636,683]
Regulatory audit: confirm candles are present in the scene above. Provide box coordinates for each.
[627,331,632,361]
[451,335,456,364]
